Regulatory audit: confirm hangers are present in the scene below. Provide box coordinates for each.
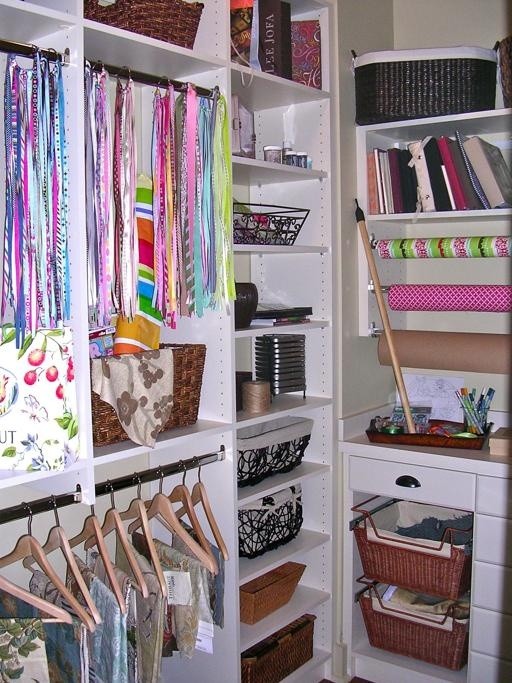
[0,454,229,619]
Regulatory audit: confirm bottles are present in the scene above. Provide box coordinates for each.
[281,140,292,165]
[263,145,282,164]
[307,158,313,169]
[296,152,309,169]
[285,150,296,167]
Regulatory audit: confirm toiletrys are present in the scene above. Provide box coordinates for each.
[264,141,312,168]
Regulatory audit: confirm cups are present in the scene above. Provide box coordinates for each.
[459,404,491,435]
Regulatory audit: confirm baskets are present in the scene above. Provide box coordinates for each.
[357,591,470,671]
[89,344,206,447]
[351,41,501,125]
[239,562,307,625]
[238,482,303,558]
[237,434,311,487]
[240,614,317,682]
[233,201,310,246]
[354,524,472,601]
[84,2,204,50]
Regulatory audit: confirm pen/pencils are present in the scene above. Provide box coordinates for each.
[455,385,495,436]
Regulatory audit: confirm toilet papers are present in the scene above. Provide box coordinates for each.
[241,380,270,413]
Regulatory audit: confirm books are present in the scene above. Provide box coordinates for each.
[366,130,512,214]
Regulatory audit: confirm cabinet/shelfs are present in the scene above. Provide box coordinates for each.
[338,432,512,683]
[226,0,346,683]
[0,0,241,683]
[354,106,512,338]
